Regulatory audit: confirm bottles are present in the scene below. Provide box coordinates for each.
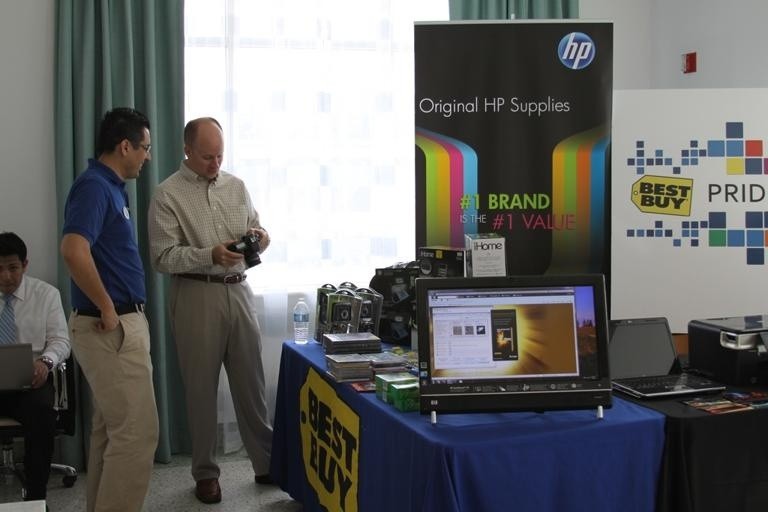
[293,299,311,345]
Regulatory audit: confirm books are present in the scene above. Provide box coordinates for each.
[322,330,407,384]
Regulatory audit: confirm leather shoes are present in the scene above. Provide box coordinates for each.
[196,478,222,505]
[254,474,282,486]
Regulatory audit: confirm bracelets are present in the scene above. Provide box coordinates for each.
[38,353,55,372]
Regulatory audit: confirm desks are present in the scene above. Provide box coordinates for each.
[268,341,669,511]
[610,384,768,511]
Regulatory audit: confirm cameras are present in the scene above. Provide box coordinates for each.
[319,292,373,324]
[227,233,262,268]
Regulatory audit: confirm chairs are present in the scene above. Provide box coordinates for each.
[1,348,79,501]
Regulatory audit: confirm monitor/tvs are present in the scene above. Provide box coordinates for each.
[415,273,613,426]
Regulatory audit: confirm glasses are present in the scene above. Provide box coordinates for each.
[126,138,152,153]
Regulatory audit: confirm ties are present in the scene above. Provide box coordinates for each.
[0,294,17,346]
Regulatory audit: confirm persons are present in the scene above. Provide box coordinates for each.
[0,230,73,512]
[144,117,295,506]
[69,109,161,511]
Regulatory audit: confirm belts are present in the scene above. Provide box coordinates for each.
[72,303,146,318]
[174,271,247,284]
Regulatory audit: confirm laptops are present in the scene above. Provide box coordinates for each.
[607,317,728,399]
[0,343,34,390]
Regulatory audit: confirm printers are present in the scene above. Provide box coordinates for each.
[688,315,768,385]
